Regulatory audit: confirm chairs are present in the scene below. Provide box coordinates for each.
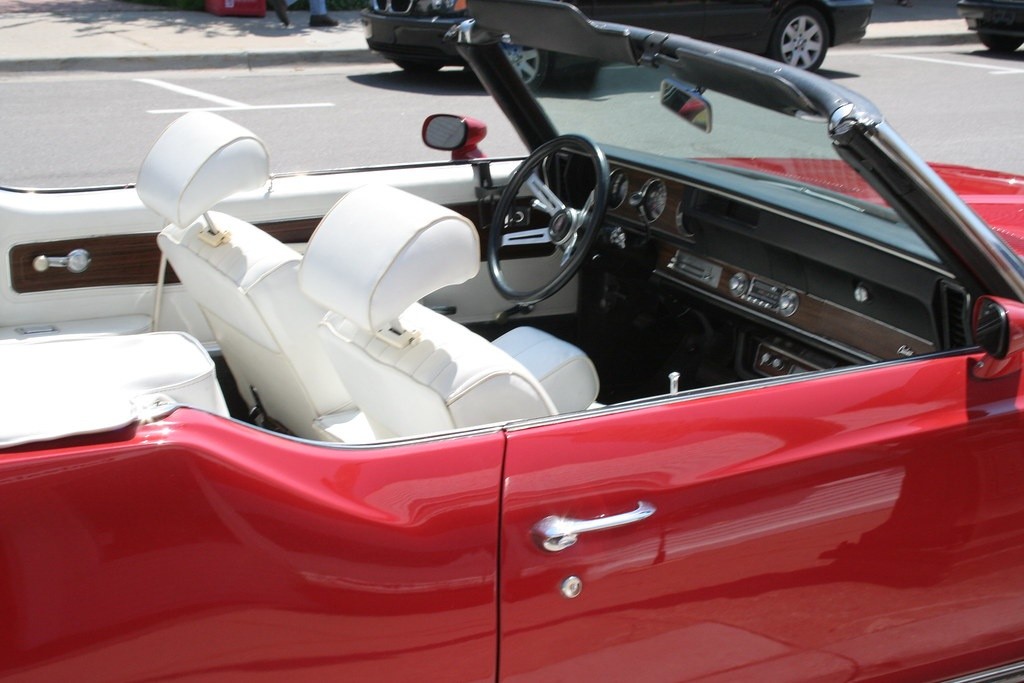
[136,109,610,440]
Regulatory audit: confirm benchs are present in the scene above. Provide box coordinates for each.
[0,331,230,450]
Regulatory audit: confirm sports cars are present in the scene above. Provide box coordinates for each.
[1,0,1024,683]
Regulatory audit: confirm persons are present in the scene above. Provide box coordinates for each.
[893,0,914,8]
[272,0,339,27]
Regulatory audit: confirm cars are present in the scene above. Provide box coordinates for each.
[954,0,1023,56]
[356,0,874,94]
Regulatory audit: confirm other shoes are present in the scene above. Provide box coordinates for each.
[310,13,338,27]
[269,0,289,25]
